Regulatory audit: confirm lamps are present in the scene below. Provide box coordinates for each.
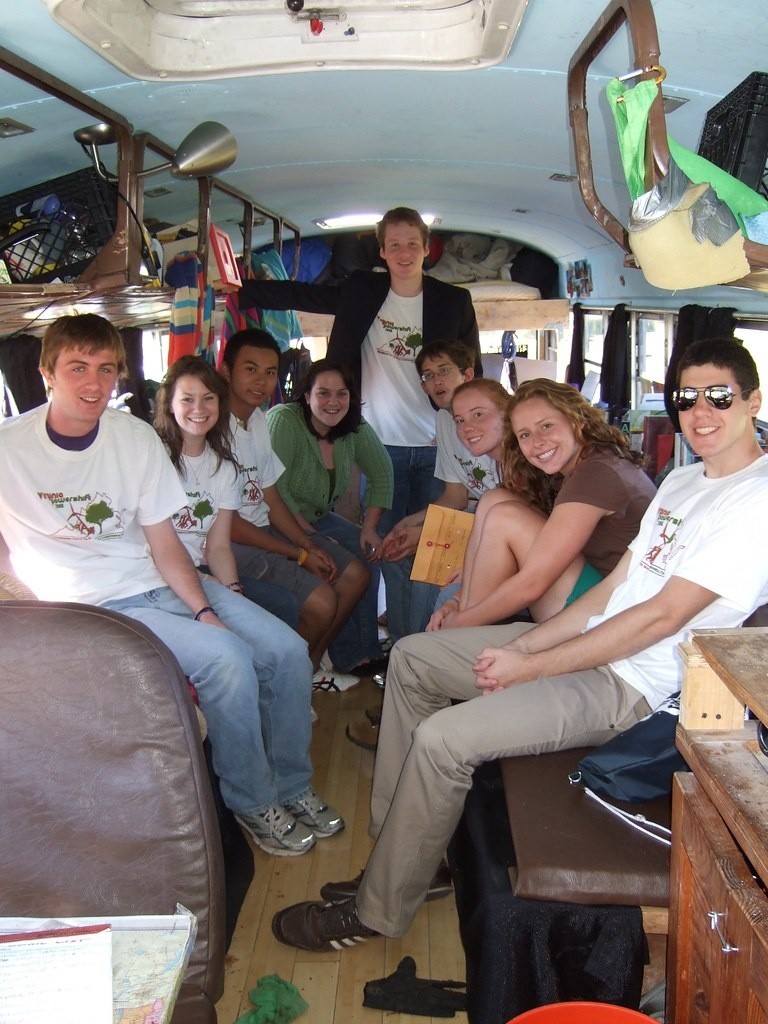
[72,120,238,184]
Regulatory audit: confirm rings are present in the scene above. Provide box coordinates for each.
[371,548,376,552]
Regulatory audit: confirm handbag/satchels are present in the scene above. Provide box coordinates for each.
[566,689,688,851]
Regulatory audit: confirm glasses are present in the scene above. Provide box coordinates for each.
[420,368,465,382]
[671,384,756,411]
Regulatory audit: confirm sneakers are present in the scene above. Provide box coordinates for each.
[271,900,380,953]
[319,856,453,903]
[310,661,360,693]
[283,785,345,838]
[234,804,317,856]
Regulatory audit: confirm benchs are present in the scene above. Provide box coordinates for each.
[502,752,673,1024]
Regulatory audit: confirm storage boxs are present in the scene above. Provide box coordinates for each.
[0,165,119,284]
[155,222,242,295]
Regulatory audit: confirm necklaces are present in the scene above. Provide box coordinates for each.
[182,443,206,485]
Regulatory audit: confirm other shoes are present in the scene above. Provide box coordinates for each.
[345,703,382,750]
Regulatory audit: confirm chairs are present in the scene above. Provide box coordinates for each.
[0,600,225,1024]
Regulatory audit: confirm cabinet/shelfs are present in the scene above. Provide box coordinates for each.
[665,627,768,1024]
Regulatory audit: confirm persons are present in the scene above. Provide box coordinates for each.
[271,338,768,953]
[237,207,483,675]
[152,324,658,674]
[0,312,346,855]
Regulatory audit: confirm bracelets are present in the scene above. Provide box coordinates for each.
[297,544,308,566]
[447,596,459,604]
[225,581,245,595]
[195,607,218,621]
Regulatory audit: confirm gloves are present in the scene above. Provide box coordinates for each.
[363,957,466,1018]
